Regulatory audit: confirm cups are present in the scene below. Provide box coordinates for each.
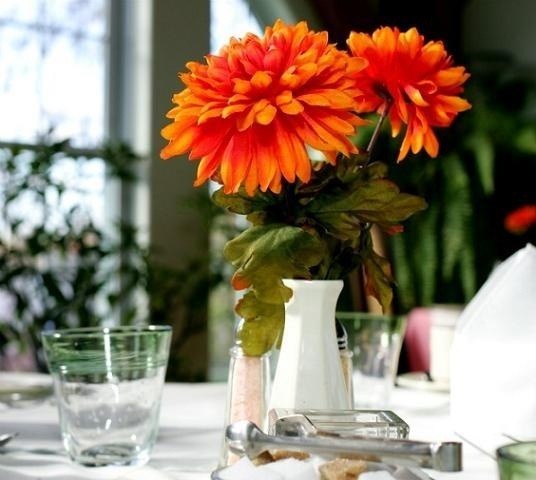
[335,311,408,411]
[42,324,173,467]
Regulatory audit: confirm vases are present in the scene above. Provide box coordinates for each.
[268,271,351,429]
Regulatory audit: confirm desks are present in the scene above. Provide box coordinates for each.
[0,361,536,480]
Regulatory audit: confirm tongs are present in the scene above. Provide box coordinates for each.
[223,418,463,473]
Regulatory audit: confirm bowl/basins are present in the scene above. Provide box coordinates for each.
[495,441,535,480]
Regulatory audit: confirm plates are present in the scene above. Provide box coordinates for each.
[211,458,433,480]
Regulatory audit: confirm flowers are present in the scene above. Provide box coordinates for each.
[152,21,474,361]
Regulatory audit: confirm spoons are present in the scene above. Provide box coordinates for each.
[1,430,20,449]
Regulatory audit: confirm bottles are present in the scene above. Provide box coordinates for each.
[220,344,273,465]
